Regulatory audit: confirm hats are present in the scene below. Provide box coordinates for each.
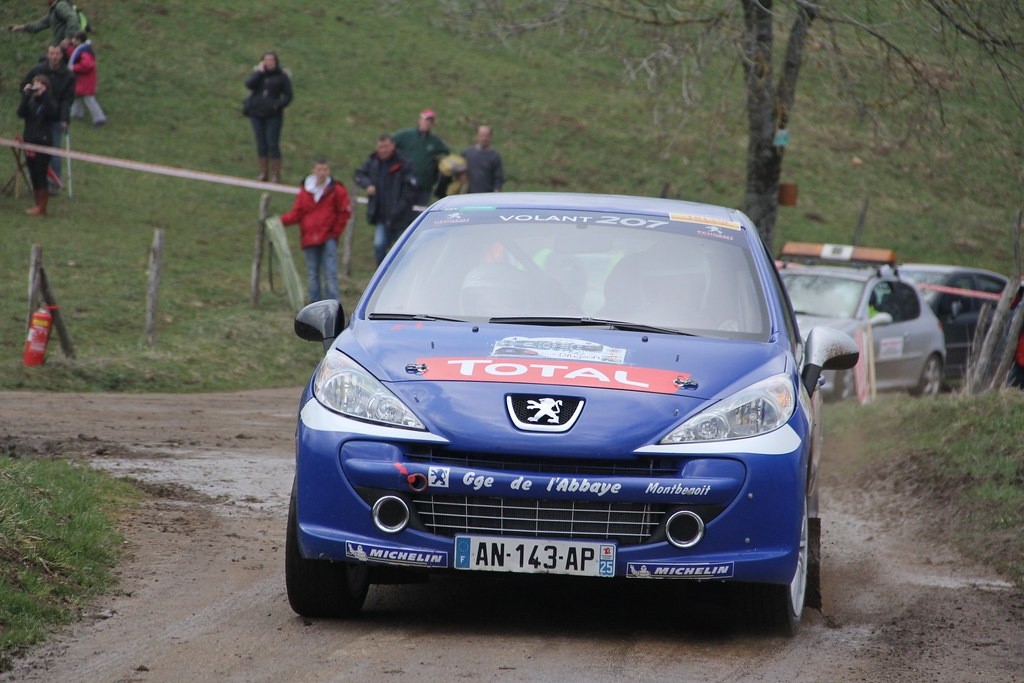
[420,110,435,120]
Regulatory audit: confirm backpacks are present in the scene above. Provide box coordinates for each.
[71,4,88,35]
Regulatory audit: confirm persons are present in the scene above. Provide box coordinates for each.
[459,126,504,192]
[281,157,351,302]
[391,110,451,207]
[243,52,292,183]
[355,132,415,268]
[6,0,107,218]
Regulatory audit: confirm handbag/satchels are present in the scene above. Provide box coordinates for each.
[244,94,252,116]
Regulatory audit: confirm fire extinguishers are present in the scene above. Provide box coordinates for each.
[23,306,58,366]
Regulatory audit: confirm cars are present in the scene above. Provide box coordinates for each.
[882,261,1024,395]
[282,193,861,629]
[747,242,950,407]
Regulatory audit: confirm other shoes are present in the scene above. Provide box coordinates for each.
[94,119,105,128]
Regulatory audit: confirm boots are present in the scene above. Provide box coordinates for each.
[258,156,268,181]
[270,159,281,183]
[23,186,48,215]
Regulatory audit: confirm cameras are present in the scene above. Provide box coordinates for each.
[27,88,39,95]
